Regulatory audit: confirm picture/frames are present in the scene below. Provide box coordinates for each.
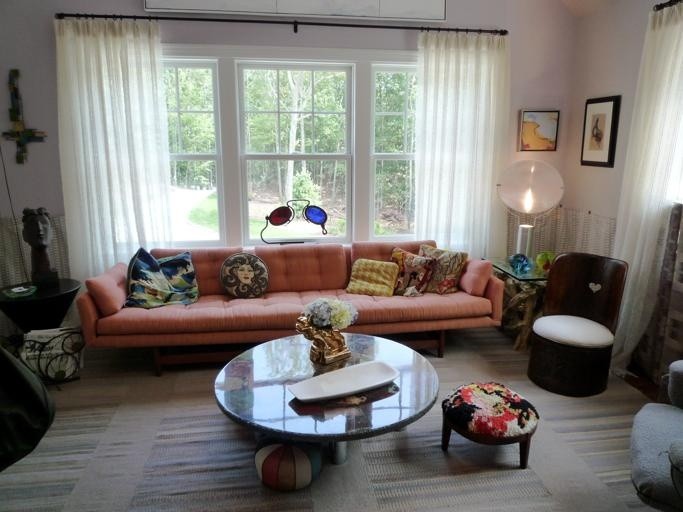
[581,95,621,168]
[518,109,560,151]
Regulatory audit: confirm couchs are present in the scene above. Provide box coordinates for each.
[631,360,683,509]
[79,240,505,369]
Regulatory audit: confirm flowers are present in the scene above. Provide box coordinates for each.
[303,297,358,329]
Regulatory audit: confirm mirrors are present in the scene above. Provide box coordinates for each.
[496,159,565,255]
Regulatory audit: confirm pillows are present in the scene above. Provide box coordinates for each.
[459,259,492,295]
[220,253,270,297]
[345,258,399,297]
[123,247,199,308]
[84,261,134,314]
[391,246,436,296]
[418,244,468,293]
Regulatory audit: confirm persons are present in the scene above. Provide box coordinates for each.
[23,207,56,273]
[223,254,267,297]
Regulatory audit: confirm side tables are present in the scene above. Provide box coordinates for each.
[482,256,547,351]
[0,279,81,333]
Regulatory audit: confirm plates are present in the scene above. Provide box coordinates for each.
[288,360,400,403]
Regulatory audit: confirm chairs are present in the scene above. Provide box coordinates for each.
[527,252,628,397]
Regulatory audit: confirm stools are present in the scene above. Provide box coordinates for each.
[440,383,537,469]
[254,442,322,490]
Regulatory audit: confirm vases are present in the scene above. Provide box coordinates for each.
[295,316,351,365]
